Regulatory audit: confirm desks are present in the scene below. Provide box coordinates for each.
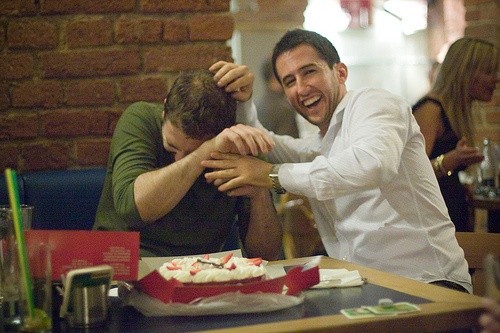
[0,254,484,333]
[466,195,500,231]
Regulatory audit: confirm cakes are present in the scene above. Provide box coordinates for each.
[156,253,266,286]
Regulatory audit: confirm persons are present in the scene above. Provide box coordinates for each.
[411,35,500,233]
[202,28,474,295]
[257,59,300,139]
[91,68,283,262]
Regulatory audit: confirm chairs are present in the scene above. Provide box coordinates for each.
[456,232,500,297]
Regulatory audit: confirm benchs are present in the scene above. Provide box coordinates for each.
[0,168,106,230]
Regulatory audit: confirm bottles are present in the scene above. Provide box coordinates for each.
[477,137,498,197]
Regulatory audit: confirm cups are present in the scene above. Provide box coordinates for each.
[69,281,109,329]
[0,204,53,333]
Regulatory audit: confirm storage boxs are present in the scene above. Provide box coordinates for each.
[7,231,141,280]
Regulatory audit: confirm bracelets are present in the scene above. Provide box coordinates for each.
[269,163,286,194]
[434,154,448,176]
